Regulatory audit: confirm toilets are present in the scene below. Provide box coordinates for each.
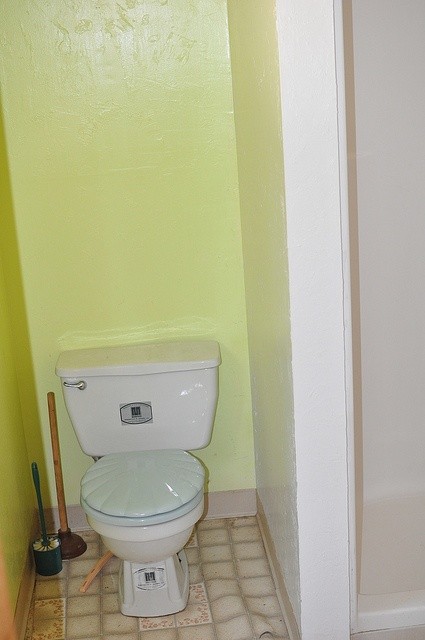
[55,341,222,618]
